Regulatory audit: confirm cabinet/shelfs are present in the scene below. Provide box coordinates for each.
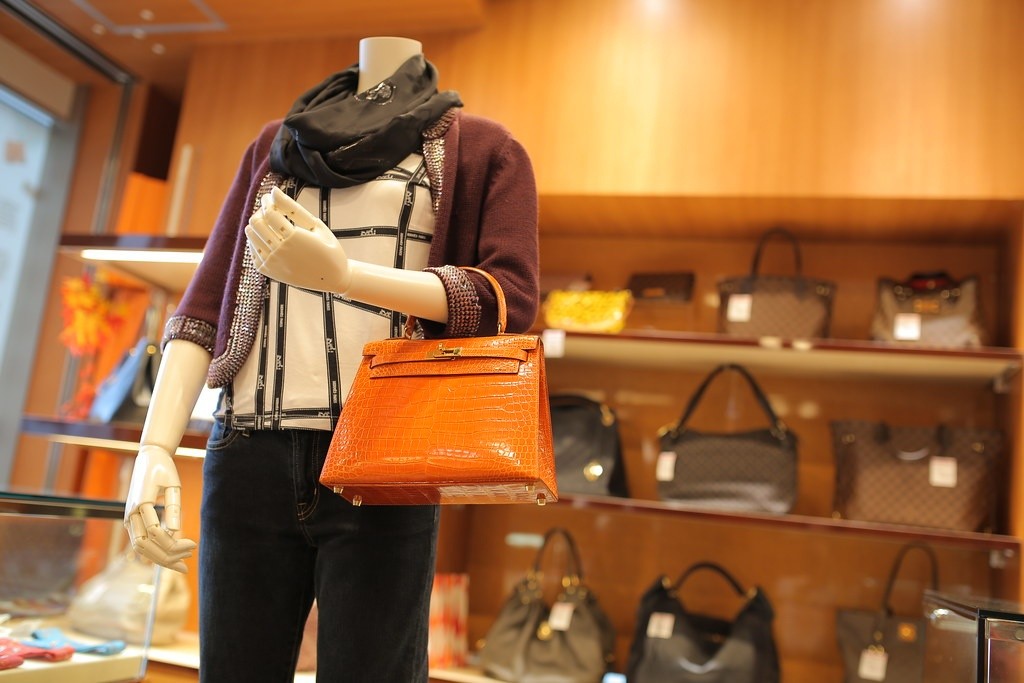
[1,229,1024,683]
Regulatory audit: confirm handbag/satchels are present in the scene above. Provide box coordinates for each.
[130,302,161,406]
[469,527,615,683]
[868,270,988,349]
[653,363,796,513]
[714,227,836,338]
[319,266,560,505]
[544,392,629,499]
[626,271,694,300]
[543,288,634,335]
[626,562,779,683]
[835,542,939,683]
[830,422,1009,534]
[65,526,188,648]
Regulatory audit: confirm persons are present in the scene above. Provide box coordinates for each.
[125,36,539,683]
